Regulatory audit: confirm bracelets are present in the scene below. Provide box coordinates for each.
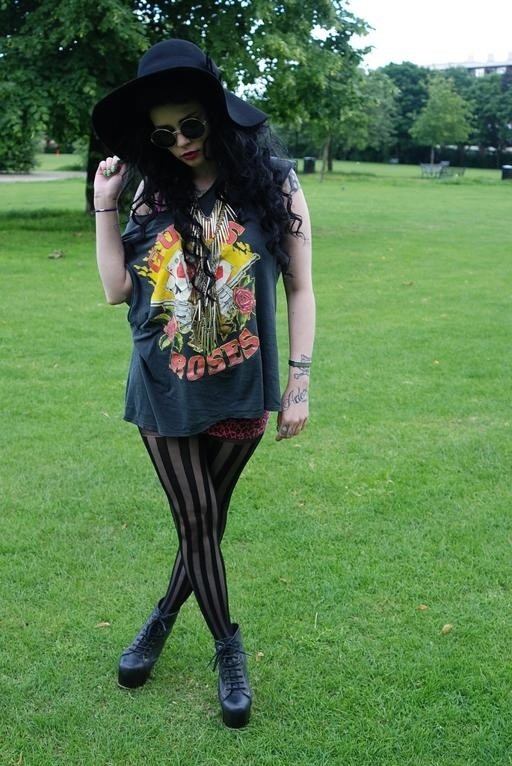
[95,208,118,213]
[289,360,312,368]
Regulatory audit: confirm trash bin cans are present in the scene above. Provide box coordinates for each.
[502,165,512,181]
[303,156,314,174]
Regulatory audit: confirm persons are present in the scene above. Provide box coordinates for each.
[89,39,318,732]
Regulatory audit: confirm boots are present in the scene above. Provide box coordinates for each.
[116,599,179,689]
[204,623,252,728]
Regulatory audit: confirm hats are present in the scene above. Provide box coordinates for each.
[91,39,268,161]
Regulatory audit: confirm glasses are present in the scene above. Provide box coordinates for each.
[149,117,206,150]
